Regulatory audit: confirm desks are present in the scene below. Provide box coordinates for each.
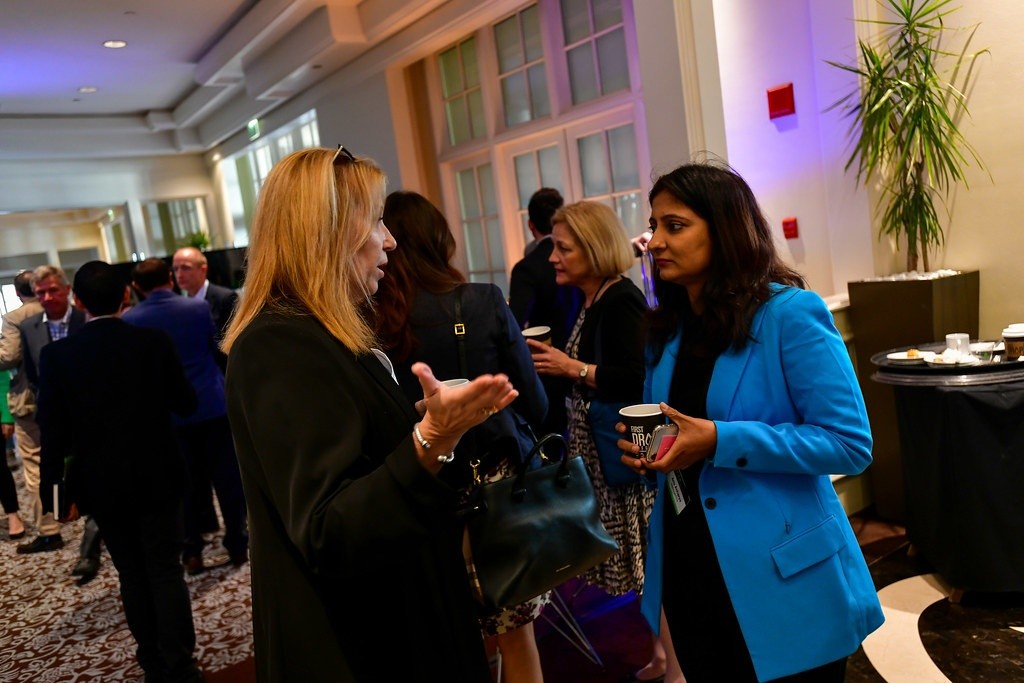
[892,381,1024,612]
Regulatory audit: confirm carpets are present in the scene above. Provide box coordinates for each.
[0,466,256,683]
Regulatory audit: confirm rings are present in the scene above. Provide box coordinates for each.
[479,405,500,416]
[539,362,542,368]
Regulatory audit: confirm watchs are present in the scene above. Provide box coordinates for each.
[579,363,589,384]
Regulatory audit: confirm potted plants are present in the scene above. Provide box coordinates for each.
[820,0,994,528]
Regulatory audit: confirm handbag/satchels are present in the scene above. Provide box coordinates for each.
[456,432,618,610]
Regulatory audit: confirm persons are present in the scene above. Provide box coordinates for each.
[0,265,132,580]
[219,145,519,683]
[631,232,652,258]
[508,188,585,382]
[120,258,250,575]
[172,246,247,534]
[615,165,885,683]
[526,200,686,683]
[31,261,205,683]
[360,192,549,683]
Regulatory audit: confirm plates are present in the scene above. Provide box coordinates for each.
[440,379,471,389]
[886,351,935,360]
[924,355,978,364]
[968,342,1006,353]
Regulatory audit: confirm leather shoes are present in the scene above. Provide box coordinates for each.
[17,533,64,553]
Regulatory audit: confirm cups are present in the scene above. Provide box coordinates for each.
[619,402,667,458]
[1002,322,1024,360]
[521,326,553,361]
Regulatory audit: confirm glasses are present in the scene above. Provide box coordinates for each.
[333,143,355,165]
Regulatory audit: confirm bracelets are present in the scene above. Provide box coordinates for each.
[414,422,454,464]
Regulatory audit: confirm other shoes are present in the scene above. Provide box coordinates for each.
[181,552,202,576]
[8,518,23,537]
[73,558,98,573]
[223,529,249,563]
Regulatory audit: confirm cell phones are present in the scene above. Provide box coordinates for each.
[647,424,679,463]
